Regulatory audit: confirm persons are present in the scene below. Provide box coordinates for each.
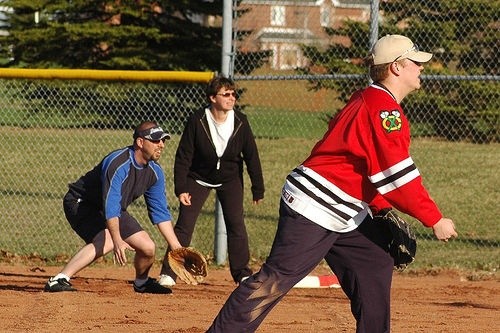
[43,123,207,293]
[206,34,458,333]
[159,77,265,283]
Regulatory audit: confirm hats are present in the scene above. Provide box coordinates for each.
[370,34,433,65]
[134,126,170,141]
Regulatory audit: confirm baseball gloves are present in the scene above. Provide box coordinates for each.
[167,246,208,286]
[358,211,417,272]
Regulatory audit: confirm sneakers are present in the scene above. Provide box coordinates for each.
[44,277,77,292]
[133,277,172,293]
[158,274,176,286]
[237,276,250,286]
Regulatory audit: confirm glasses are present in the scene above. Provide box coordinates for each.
[216,93,236,97]
[393,44,418,63]
[145,138,165,143]
